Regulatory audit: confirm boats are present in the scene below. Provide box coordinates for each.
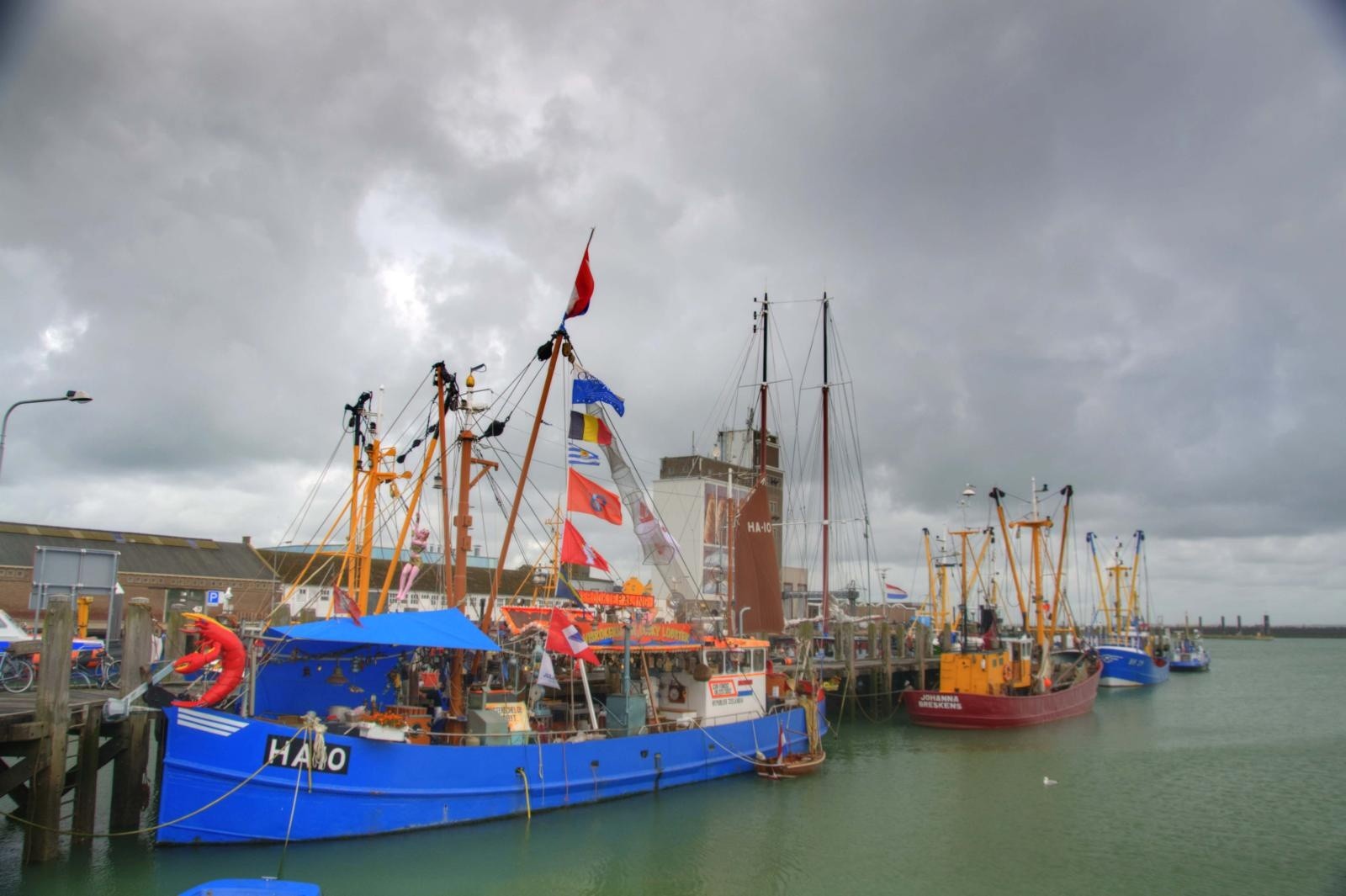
[1167,614,1211,671]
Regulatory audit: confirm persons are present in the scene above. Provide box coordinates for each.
[396,513,429,600]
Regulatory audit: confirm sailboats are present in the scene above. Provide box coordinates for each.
[105,313,834,850]
[900,475,1108,727]
[1051,529,1173,691]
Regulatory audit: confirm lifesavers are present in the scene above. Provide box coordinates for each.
[1003,664,1012,681]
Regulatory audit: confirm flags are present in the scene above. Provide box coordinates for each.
[559,249,595,326]
[567,466,622,525]
[545,606,600,666]
[568,410,612,445]
[556,565,586,608]
[560,518,610,573]
[568,442,600,466]
[572,379,625,417]
[536,651,561,690]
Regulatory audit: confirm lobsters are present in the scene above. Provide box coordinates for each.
[170,611,248,708]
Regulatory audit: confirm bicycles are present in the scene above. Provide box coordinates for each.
[69,650,123,691]
[0,647,34,693]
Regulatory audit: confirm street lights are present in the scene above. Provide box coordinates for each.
[0,390,95,470]
